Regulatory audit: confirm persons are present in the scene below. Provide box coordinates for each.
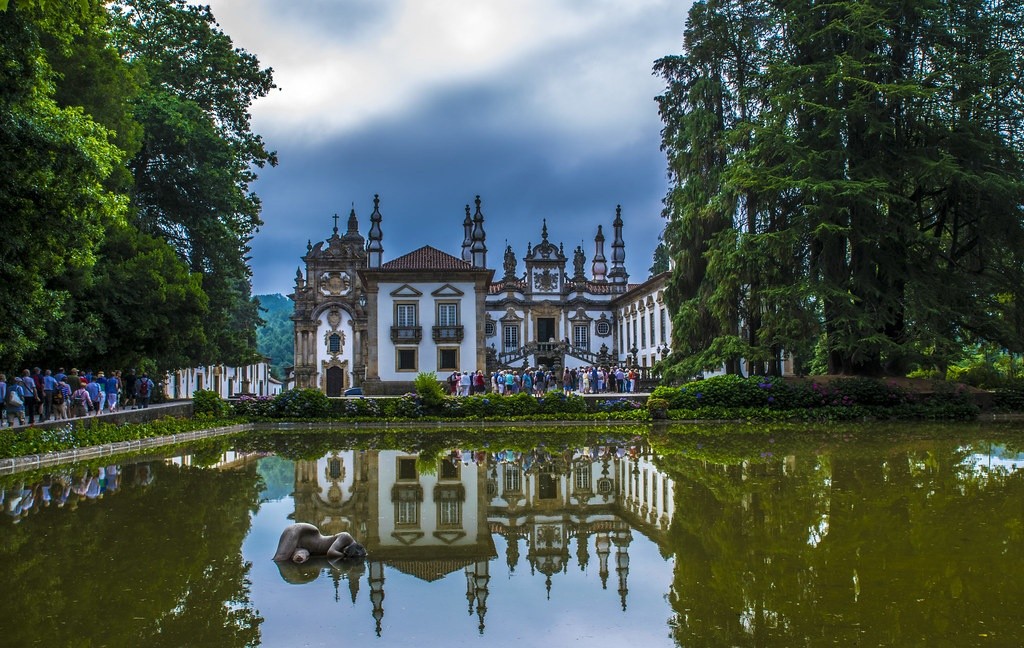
[0,462,157,524]
[448,366,640,397]
[0,367,155,427]
[448,440,642,476]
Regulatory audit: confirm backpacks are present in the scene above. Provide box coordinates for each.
[75,390,86,406]
[140,378,149,395]
[53,384,64,404]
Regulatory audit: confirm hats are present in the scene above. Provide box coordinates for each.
[14,377,22,384]
[33,367,41,373]
[71,368,79,372]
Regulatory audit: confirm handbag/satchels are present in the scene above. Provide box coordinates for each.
[42,389,46,398]
[36,397,40,401]
[10,386,24,406]
